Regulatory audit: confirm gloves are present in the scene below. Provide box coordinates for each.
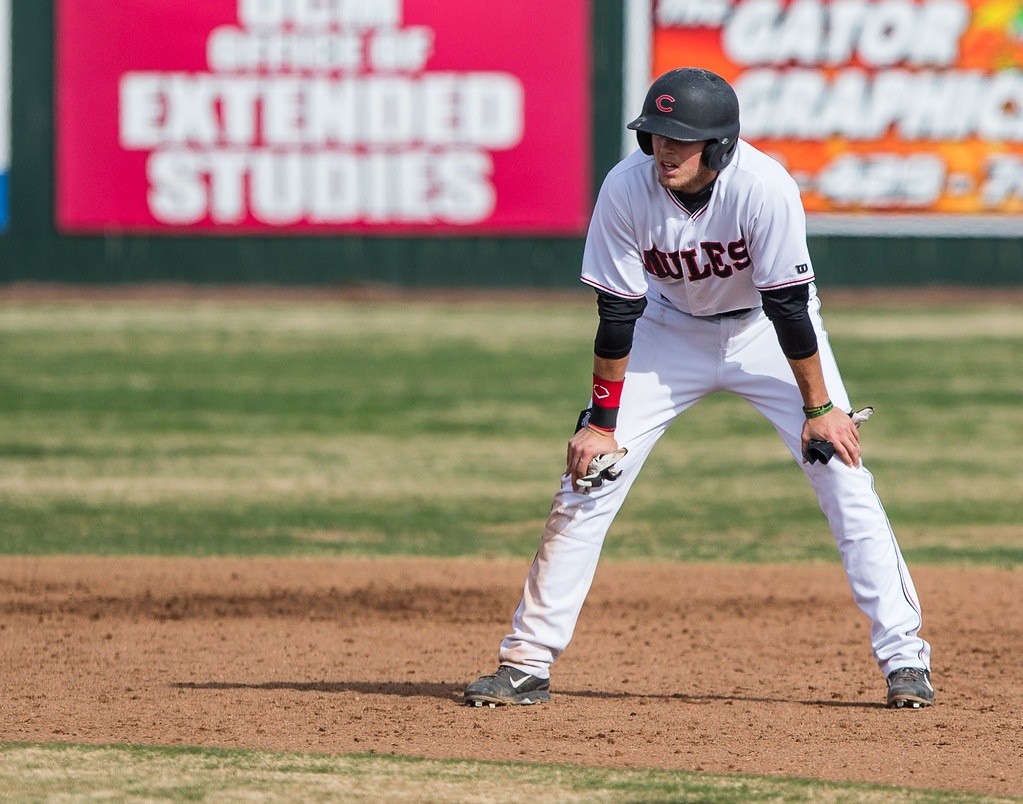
[804,406,875,465]
[571,407,628,494]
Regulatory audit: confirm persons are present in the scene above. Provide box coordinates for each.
[463,67,935,708]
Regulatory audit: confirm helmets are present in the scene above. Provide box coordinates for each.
[627,67,741,171]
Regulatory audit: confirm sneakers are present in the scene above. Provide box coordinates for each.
[886,667,935,708]
[464,666,550,708]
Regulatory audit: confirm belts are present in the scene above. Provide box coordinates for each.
[660,294,754,318]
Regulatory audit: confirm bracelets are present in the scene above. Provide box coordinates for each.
[802,400,833,417]
